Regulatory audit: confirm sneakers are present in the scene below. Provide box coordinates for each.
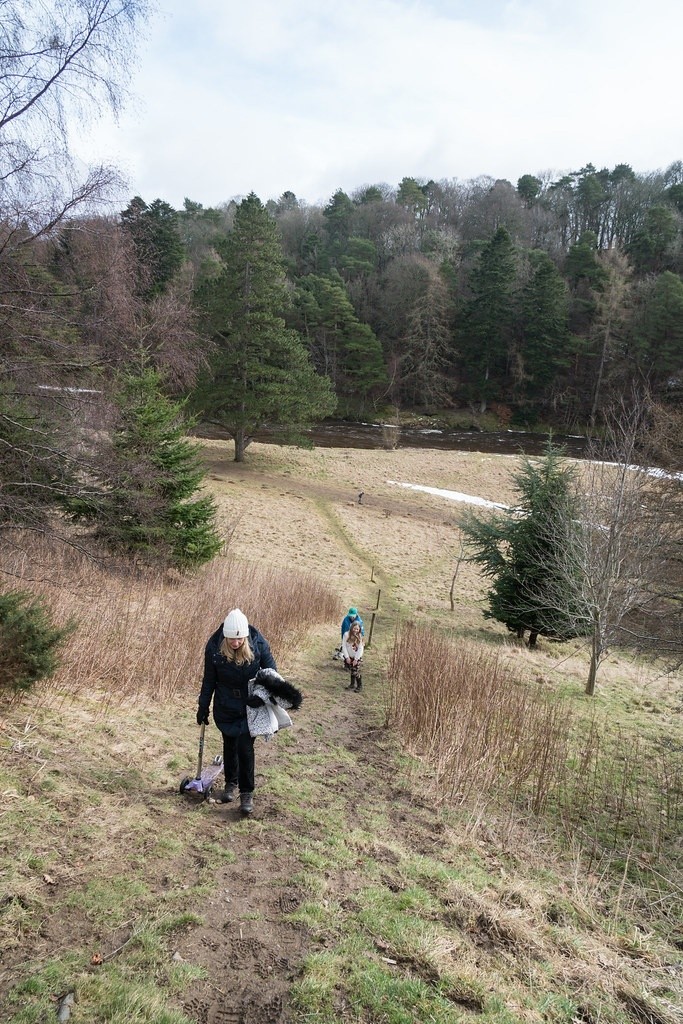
[236,792,254,813]
[221,781,239,802]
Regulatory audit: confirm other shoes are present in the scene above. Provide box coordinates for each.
[344,662,349,669]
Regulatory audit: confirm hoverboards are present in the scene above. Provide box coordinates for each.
[333,646,343,660]
[180,716,224,800]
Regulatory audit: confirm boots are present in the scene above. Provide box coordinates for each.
[354,675,363,693]
[345,675,355,690]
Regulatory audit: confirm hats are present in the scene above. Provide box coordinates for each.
[223,608,249,639]
[347,608,358,617]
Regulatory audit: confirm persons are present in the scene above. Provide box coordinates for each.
[333,607,364,660]
[197,610,277,813]
[342,621,364,692]
[358,492,365,503]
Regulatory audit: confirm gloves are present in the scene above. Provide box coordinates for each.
[196,705,210,725]
[244,694,263,708]
